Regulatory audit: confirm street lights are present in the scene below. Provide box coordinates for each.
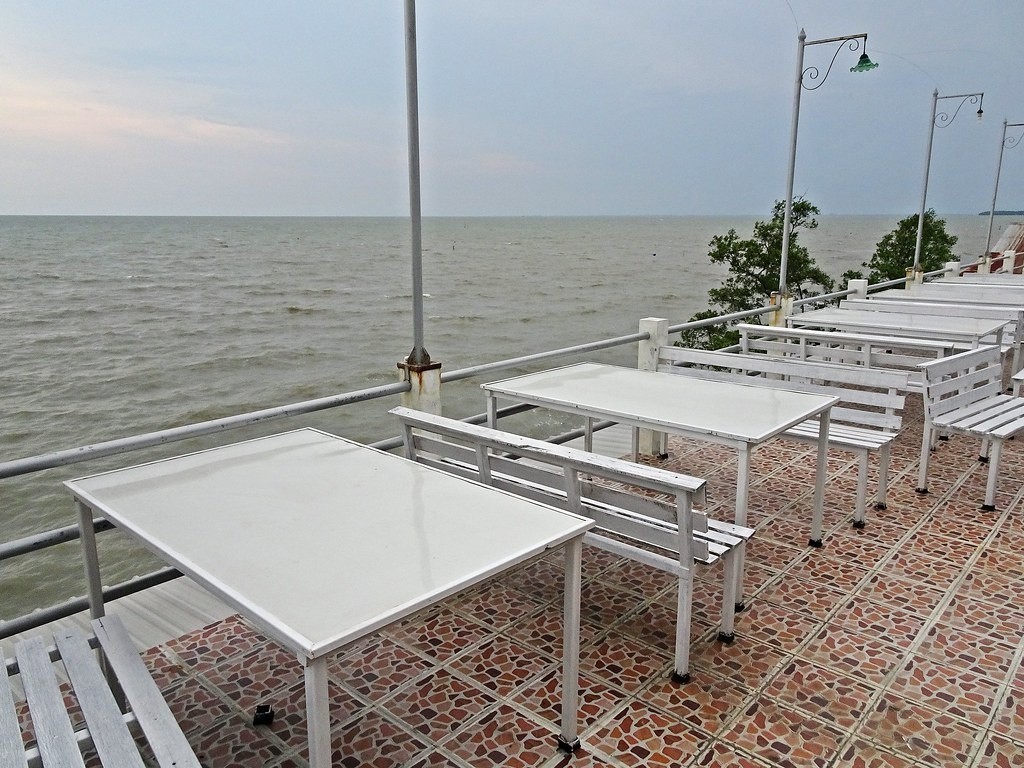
[777,25,880,294]
[912,87,984,266]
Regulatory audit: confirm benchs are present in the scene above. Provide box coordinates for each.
[0,264,1024,768]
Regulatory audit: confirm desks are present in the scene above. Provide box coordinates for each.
[931,275,1024,283]
[779,308,1012,446]
[478,359,839,612]
[862,285,1024,392]
[61,422,609,768]
